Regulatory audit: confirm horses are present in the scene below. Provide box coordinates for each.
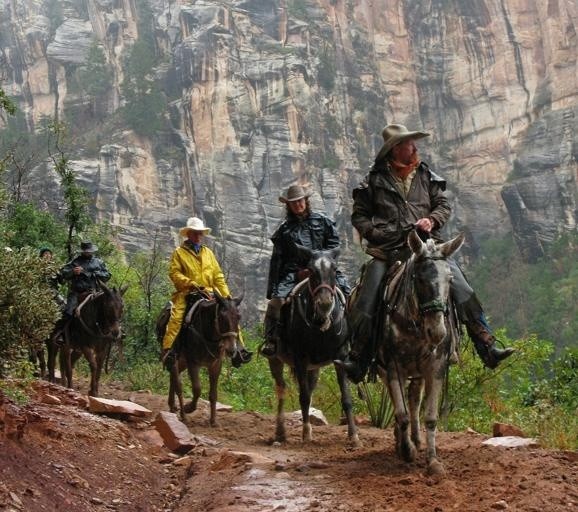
[348,226,467,476]
[263,240,360,451]
[158,282,245,433]
[27,282,130,402]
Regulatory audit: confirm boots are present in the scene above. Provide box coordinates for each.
[262,314,280,356]
[56,311,73,344]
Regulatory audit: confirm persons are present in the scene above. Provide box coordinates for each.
[157,217,253,370]
[345,123,516,381]
[261,184,351,355]
[40,248,64,306]
[57,240,128,344]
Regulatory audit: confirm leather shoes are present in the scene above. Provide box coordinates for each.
[479,346,516,369]
[333,359,368,384]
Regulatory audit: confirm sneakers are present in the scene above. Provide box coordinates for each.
[232,351,253,368]
[160,350,178,361]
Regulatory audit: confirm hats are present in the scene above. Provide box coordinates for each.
[179,217,212,237]
[279,185,314,203]
[39,247,52,257]
[376,124,431,164]
[75,239,98,253]
